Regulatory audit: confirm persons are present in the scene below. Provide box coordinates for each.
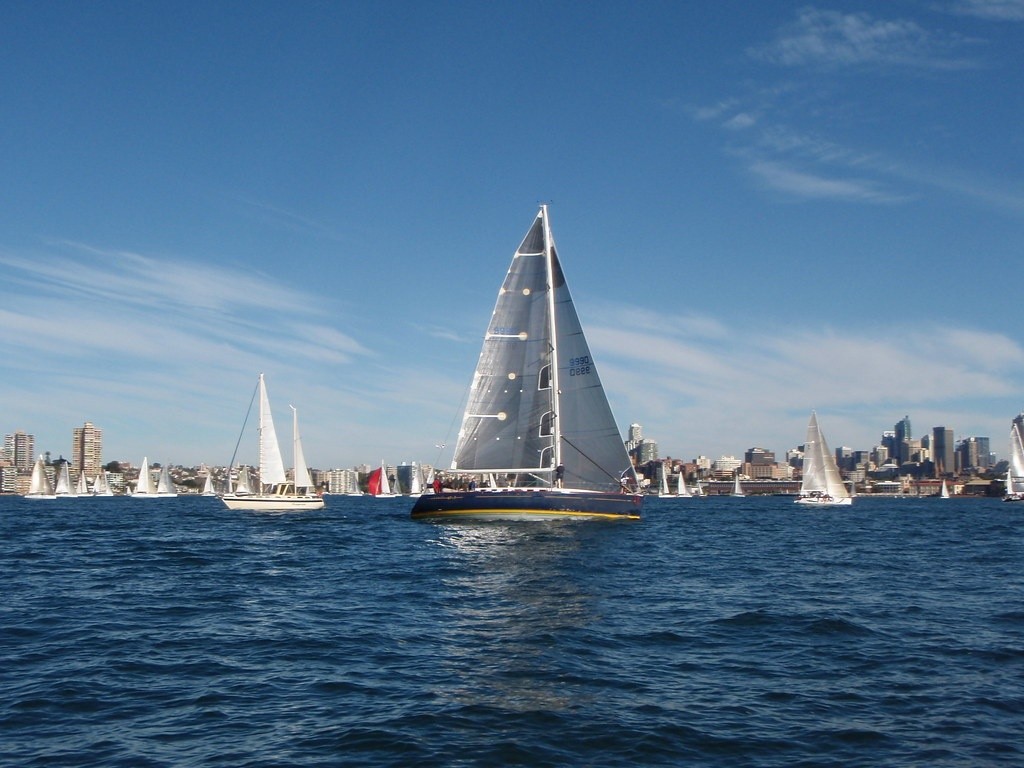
[432,476,442,493]
[507,472,517,488]
[553,463,565,489]
[469,478,475,492]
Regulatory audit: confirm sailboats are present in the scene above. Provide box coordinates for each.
[730,475,745,498]
[793,408,854,506]
[221,372,326,512]
[53,460,79,498]
[1001,468,1021,502]
[75,470,94,497]
[201,474,217,498]
[657,465,678,499]
[677,472,694,498]
[410,204,646,524]
[124,457,179,499]
[347,463,403,498]
[94,474,114,497]
[23,454,58,501]
[939,479,951,499]
